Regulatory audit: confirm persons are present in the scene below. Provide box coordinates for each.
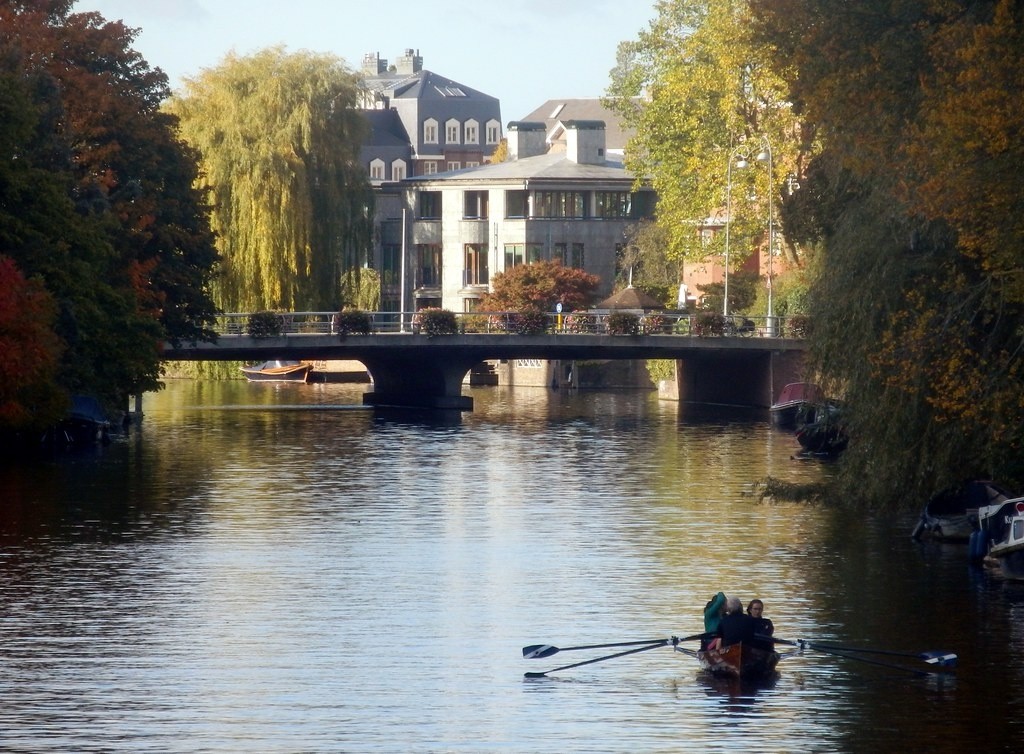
[703,592,727,650]
[968,472,991,558]
[747,599,773,650]
[716,596,755,649]
[121,411,132,439]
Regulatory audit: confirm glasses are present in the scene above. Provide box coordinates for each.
[752,608,762,610]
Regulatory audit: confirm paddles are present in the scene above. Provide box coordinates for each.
[522,634,703,659]
[524,635,702,680]
[774,637,956,667]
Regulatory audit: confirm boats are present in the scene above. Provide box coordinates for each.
[240,362,313,383]
[769,384,846,430]
[793,406,851,455]
[911,475,1024,581]
[695,592,777,685]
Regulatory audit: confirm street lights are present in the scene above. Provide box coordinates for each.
[724,144,750,334]
[758,134,776,338]
